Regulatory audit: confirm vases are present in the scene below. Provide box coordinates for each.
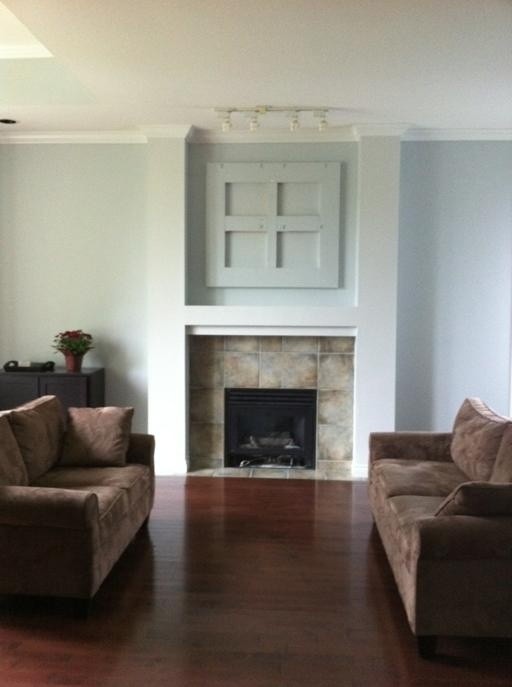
[57,346,92,371]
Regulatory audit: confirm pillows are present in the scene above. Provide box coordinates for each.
[431,474,511,517]
[60,400,136,467]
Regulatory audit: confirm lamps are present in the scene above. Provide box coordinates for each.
[208,103,336,139]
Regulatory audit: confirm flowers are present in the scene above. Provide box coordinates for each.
[49,326,96,359]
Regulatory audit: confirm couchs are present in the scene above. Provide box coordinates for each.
[365,393,511,664]
[0,391,159,625]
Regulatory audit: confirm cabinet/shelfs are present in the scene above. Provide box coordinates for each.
[0,362,108,413]
